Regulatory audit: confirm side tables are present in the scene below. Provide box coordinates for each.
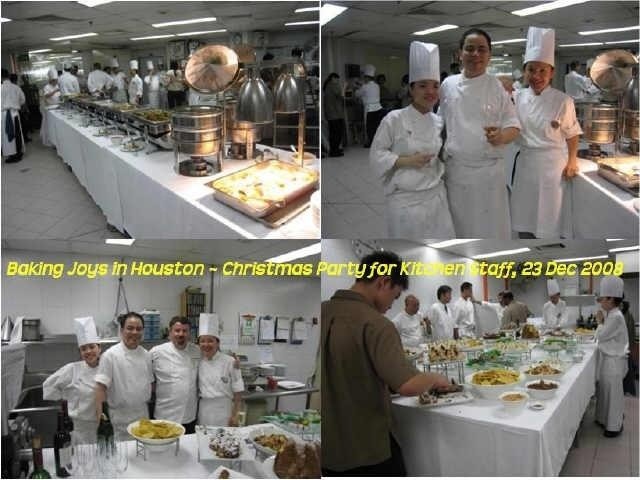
[563,139,638,240]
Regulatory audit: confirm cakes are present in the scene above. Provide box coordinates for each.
[209,429,241,457]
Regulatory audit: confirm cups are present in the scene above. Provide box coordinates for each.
[309,190,321,232]
[267,377,277,392]
[61,433,129,479]
[481,105,502,133]
[63,102,122,145]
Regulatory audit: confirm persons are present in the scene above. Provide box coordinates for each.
[436,26,522,242]
[1,57,188,163]
[323,25,596,158]
[94,312,156,440]
[371,40,458,240]
[150,316,240,435]
[514,25,582,238]
[42,316,107,444]
[197,313,245,428]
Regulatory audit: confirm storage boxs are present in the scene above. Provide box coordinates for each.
[140,309,162,342]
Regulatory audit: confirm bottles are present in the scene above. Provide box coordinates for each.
[96,401,114,457]
[53,412,72,477]
[514,319,521,341]
[28,437,53,479]
[62,400,77,455]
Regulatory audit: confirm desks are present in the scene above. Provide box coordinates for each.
[237,371,318,418]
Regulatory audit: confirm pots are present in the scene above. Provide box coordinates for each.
[591,49,637,92]
[184,43,241,94]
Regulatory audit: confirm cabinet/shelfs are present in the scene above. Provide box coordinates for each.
[178,288,206,344]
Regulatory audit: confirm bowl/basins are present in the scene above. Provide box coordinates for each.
[500,392,529,415]
[464,369,524,399]
[290,152,317,164]
[126,419,186,444]
[526,379,561,400]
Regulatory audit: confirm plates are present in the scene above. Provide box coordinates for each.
[416,392,476,407]
[531,402,544,411]
[526,369,565,378]
[278,381,307,390]
[241,363,285,379]
[120,144,145,153]
[194,423,287,479]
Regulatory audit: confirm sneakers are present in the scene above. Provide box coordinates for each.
[4,151,25,163]
[328,147,345,158]
[601,425,625,438]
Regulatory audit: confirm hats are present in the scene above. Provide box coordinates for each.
[363,63,377,77]
[521,25,556,69]
[546,279,561,297]
[598,275,625,299]
[73,315,102,348]
[407,40,441,85]
[47,55,155,80]
[198,311,221,342]
